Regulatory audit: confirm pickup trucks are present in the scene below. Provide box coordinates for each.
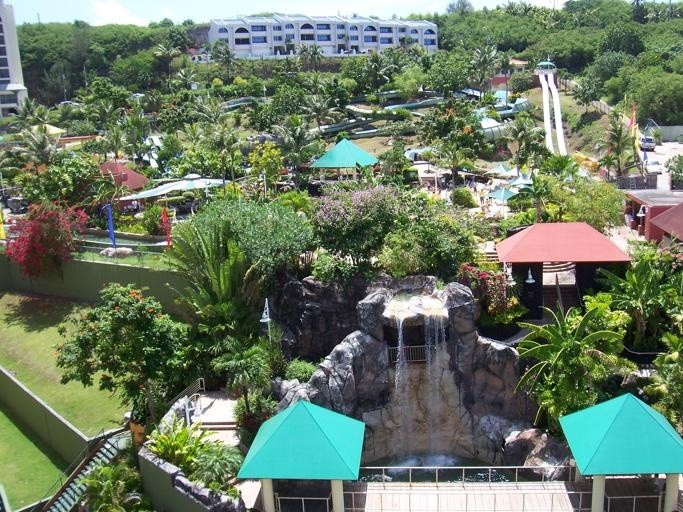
[639,136,657,152]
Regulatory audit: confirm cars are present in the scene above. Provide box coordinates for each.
[61,101,80,108]
[133,92,145,97]
[341,48,361,55]
[361,48,373,54]
[115,201,201,219]
[10,205,28,214]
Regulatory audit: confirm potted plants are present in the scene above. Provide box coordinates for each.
[129,387,168,447]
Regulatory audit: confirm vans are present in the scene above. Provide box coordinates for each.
[191,54,216,64]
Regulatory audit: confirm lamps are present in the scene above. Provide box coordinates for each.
[636,205,648,218]
[525,267,536,284]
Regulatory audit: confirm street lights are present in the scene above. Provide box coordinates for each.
[259,296,273,356]
[185,392,202,450]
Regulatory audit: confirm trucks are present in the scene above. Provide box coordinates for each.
[6,197,29,210]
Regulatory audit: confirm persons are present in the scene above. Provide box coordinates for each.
[414,162,531,215]
